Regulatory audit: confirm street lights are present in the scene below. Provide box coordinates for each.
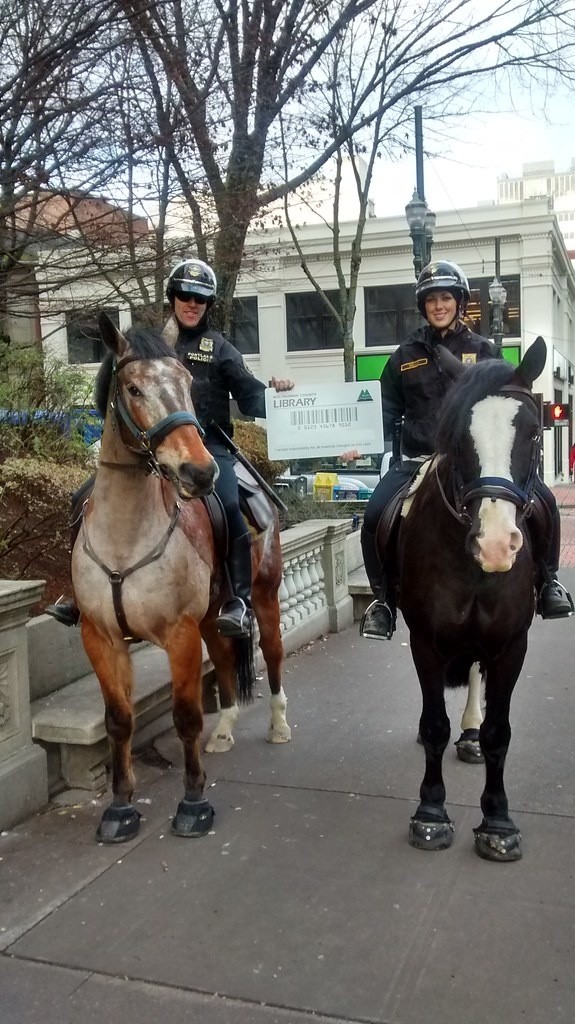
[486,234,507,354]
[405,104,436,327]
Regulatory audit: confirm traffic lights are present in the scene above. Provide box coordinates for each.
[551,404,572,420]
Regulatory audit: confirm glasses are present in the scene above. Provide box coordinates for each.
[174,289,209,305]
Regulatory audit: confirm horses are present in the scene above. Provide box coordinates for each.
[375,336,549,864]
[71,306,293,846]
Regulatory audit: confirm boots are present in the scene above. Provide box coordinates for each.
[215,530,252,638]
[360,526,396,636]
[45,593,82,625]
[534,510,571,614]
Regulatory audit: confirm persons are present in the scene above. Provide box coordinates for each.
[45,258,294,640]
[340,260,572,636]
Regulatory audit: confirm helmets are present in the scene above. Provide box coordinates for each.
[166,259,217,310]
[414,261,471,319]
